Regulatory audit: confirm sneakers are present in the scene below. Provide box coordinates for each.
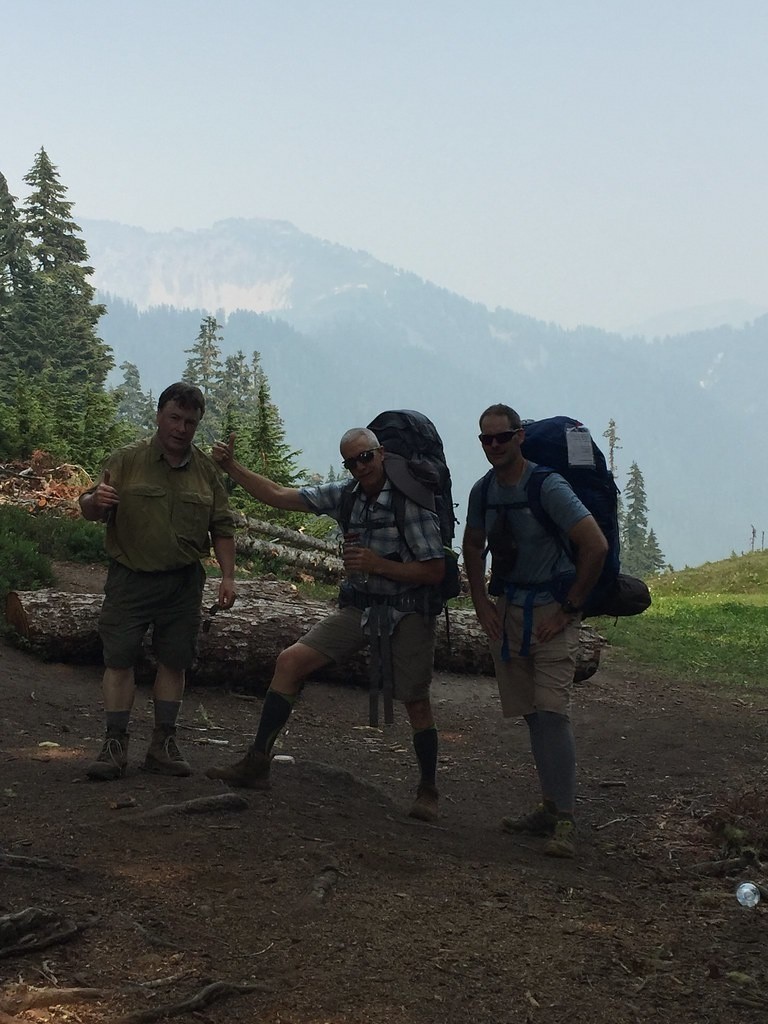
[410,783,439,819]
[85,732,129,778]
[501,805,576,834]
[543,813,578,857]
[208,753,272,788]
[143,735,191,776]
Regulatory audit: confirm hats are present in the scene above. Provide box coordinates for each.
[384,457,439,512]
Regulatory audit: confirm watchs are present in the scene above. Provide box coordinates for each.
[557,596,581,614]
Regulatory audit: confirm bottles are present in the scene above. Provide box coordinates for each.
[343,531,370,585]
[737,883,760,908]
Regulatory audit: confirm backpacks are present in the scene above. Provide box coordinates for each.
[480,415,622,612]
[479,427,519,446]
[338,412,461,601]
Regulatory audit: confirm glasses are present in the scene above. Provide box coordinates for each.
[342,447,379,469]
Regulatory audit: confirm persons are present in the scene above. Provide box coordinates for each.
[207,426,447,821]
[79,381,238,778]
[463,402,609,858]
